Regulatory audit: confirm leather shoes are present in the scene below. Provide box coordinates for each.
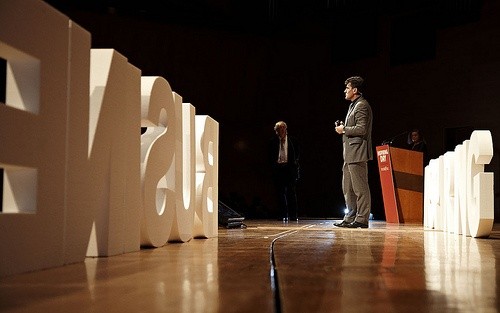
[342,220,368,228]
[333,221,350,227]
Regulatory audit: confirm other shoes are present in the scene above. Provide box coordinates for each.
[283,217,289,221]
[292,215,298,221]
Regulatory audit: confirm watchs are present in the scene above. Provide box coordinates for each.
[342,127,346,134]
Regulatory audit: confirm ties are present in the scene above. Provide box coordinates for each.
[280,138,286,162]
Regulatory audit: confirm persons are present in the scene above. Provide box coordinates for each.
[334,76,373,228]
[408,128,430,167]
[257,121,304,221]
